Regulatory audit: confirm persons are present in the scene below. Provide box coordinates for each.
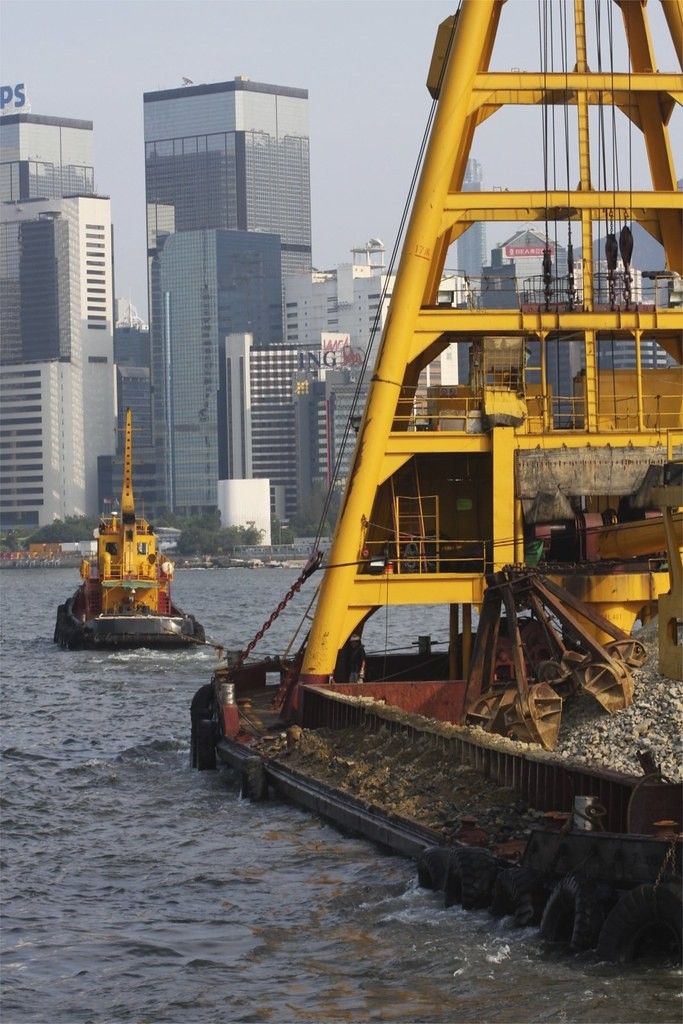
[348,633,368,684]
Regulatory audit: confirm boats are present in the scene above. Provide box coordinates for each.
[53,406,206,651]
[193,0,683,959]
